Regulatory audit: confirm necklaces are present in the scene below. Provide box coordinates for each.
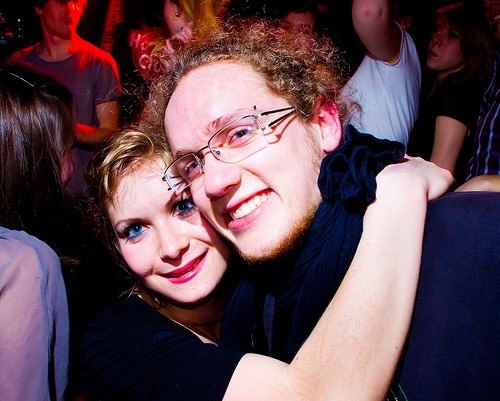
[161,304,224,326]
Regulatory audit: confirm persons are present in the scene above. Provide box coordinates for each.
[274,0,321,42]
[338,1,422,152]
[462,0,500,177]
[138,0,231,131]
[140,22,500,401]
[0,58,78,401]
[81,122,500,401]
[406,0,495,175]
[10,0,122,194]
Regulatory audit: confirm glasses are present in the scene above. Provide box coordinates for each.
[162,106,295,192]
